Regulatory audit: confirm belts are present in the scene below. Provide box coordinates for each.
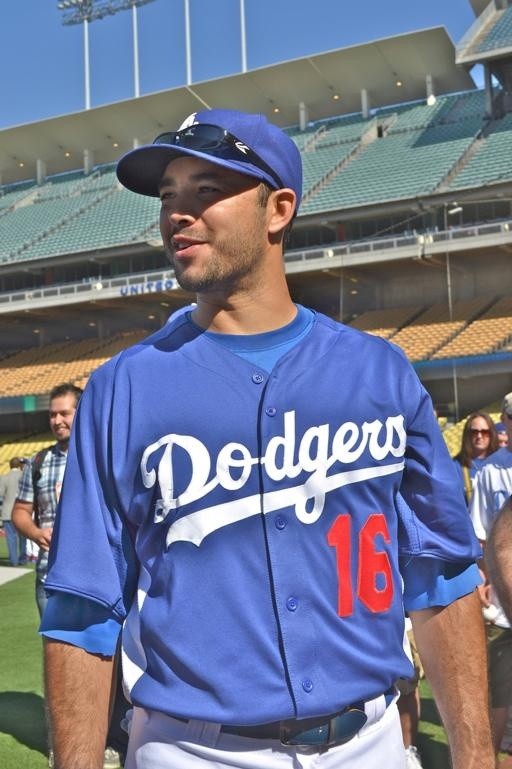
[131,684,396,750]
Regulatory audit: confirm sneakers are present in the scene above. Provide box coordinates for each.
[10,554,38,567]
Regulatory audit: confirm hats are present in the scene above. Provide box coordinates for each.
[500,392,512,414]
[115,106,304,219]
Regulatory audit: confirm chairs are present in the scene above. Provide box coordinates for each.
[1,425,59,471]
[1,288,511,400]
[473,2,512,55]
[3,83,510,269]
[430,395,507,460]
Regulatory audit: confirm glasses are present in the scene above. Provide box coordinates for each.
[468,428,492,438]
[505,412,512,419]
[151,122,285,190]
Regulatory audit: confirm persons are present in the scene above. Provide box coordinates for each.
[1,453,40,572]
[10,381,87,623]
[448,382,512,754]
[34,105,500,768]
